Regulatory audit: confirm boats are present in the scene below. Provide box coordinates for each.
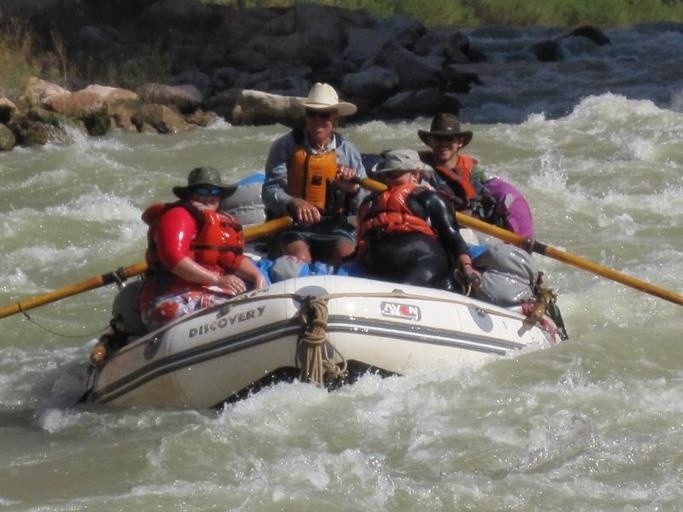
[88,256,571,406]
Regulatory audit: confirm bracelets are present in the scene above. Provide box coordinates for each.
[462,263,472,268]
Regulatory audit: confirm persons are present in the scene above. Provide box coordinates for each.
[418,115,493,224]
[356,150,482,293]
[262,83,371,265]
[140,167,267,333]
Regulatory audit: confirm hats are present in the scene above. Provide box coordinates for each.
[172,167,237,198]
[290,83,357,117]
[418,114,472,147]
[371,150,434,173]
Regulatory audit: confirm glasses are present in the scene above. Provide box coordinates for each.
[195,187,221,195]
[305,110,330,120]
